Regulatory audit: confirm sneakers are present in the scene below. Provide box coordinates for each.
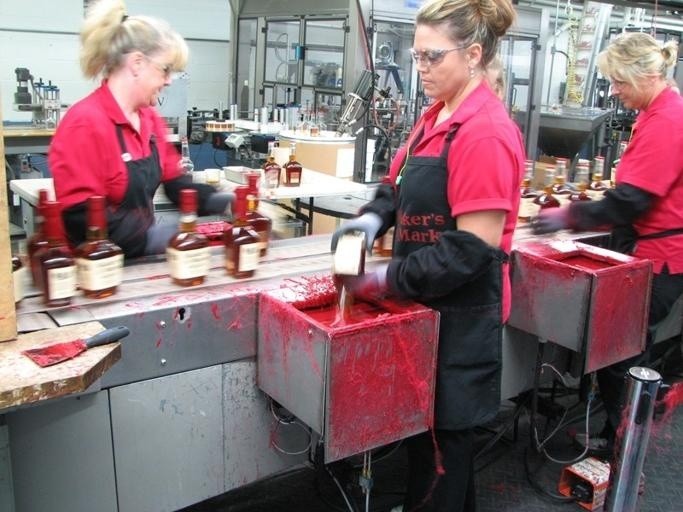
[578,431,645,495]
[392,504,404,512]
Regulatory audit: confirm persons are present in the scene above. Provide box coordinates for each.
[329,1,525,511]
[527,31,682,461]
[48,0,239,260]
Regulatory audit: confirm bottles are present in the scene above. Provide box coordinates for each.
[584,155,608,201]
[281,143,303,187]
[565,163,594,210]
[176,136,194,175]
[517,160,539,224]
[26,188,78,308]
[264,148,282,190]
[610,140,628,189]
[530,166,561,224]
[298,111,327,138]
[222,185,262,281]
[552,157,575,210]
[73,194,125,301]
[246,174,272,259]
[165,187,211,287]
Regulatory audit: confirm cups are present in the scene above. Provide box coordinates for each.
[204,168,221,192]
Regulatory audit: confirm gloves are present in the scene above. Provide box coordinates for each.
[531,208,570,234]
[331,212,381,254]
[345,264,388,301]
[145,223,178,255]
[205,192,237,213]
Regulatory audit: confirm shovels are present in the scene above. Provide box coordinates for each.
[24,326,129,369]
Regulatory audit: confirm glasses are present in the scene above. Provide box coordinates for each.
[141,53,172,74]
[409,47,466,67]
